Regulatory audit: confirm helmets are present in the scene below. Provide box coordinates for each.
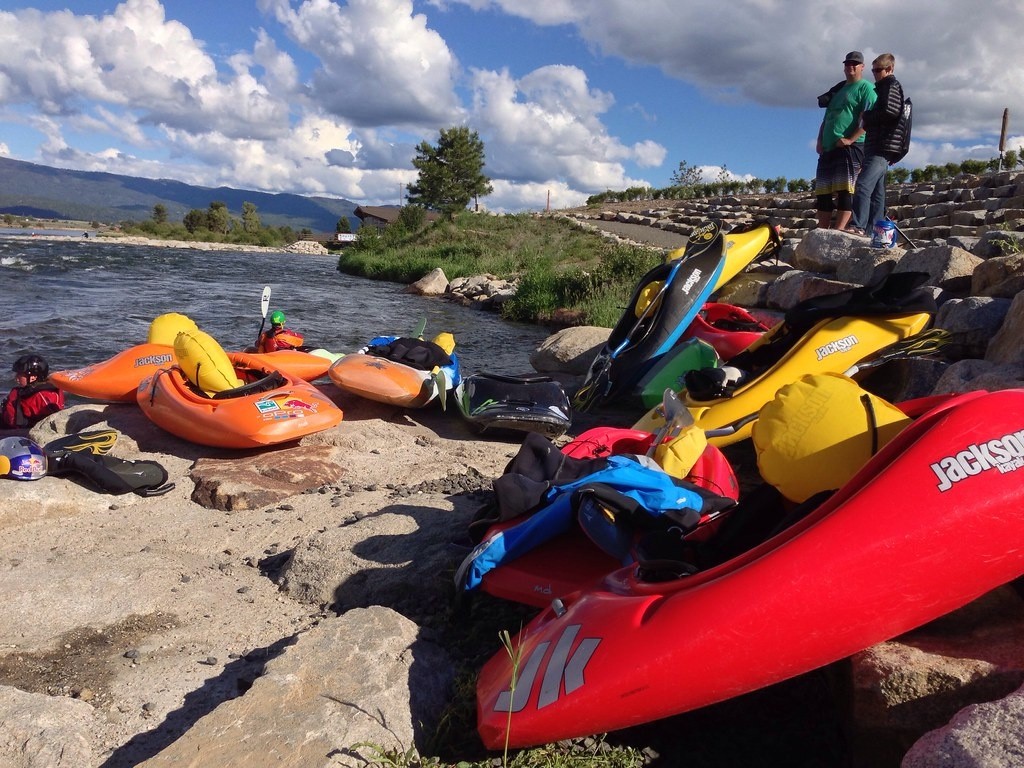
[270,310,285,324]
[0,436,47,480]
[12,355,48,377]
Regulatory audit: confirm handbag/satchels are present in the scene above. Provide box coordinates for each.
[66,452,176,496]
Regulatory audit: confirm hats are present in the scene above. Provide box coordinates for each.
[842,51,864,63]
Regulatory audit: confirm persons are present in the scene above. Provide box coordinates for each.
[0,355,64,429]
[813,51,878,236]
[255,311,303,354]
[839,53,906,236]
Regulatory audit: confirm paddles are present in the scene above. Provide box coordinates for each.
[258,286,272,337]
[573,218,724,414]
[577,388,694,561]
[704,328,954,439]
[411,317,447,413]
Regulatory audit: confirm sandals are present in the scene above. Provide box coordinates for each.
[842,226,865,236]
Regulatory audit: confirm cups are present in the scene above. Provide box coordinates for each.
[871,220,897,248]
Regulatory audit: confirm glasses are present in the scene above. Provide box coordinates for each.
[845,62,861,66]
[871,68,889,74]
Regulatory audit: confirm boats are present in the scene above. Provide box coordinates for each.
[574,216,786,421]
[335,331,464,409]
[54,313,206,406]
[138,327,346,452]
[455,365,577,440]
[224,345,351,384]
[450,257,1022,757]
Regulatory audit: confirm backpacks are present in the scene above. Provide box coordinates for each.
[894,97,912,163]
